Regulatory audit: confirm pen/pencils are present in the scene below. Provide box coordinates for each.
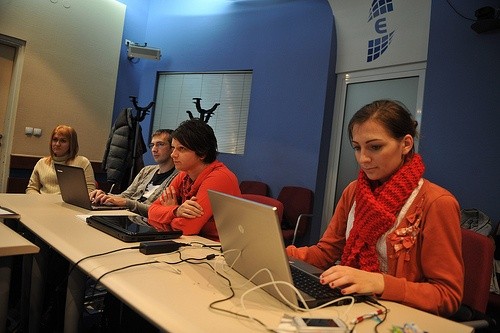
[349,309,385,324]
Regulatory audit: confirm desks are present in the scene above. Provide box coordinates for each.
[0,193,474,333]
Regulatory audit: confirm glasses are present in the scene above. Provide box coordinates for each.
[51,137,69,143]
[149,142,169,148]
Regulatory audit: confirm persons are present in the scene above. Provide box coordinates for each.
[25,125,96,195]
[148,120,243,244]
[89,129,182,216]
[286,99,465,317]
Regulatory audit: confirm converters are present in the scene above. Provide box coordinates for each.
[138,240,180,254]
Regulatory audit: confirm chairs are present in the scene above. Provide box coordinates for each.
[240,181,269,196]
[277,185,314,247]
[460,229,497,326]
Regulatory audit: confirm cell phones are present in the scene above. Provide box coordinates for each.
[293,317,346,332]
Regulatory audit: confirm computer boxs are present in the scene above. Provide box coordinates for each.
[56,278,107,333]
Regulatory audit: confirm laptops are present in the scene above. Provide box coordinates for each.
[208,189,368,310]
[86,215,183,242]
[53,163,126,210]
